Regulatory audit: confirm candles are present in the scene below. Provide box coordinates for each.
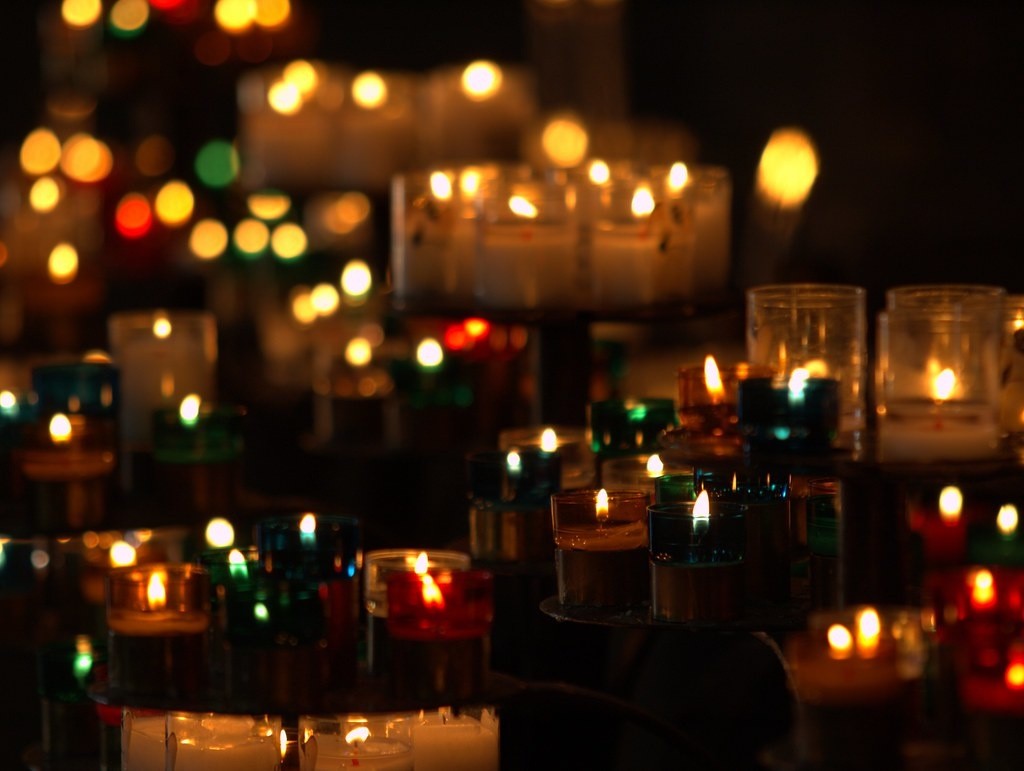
[21,60,1024,771]
